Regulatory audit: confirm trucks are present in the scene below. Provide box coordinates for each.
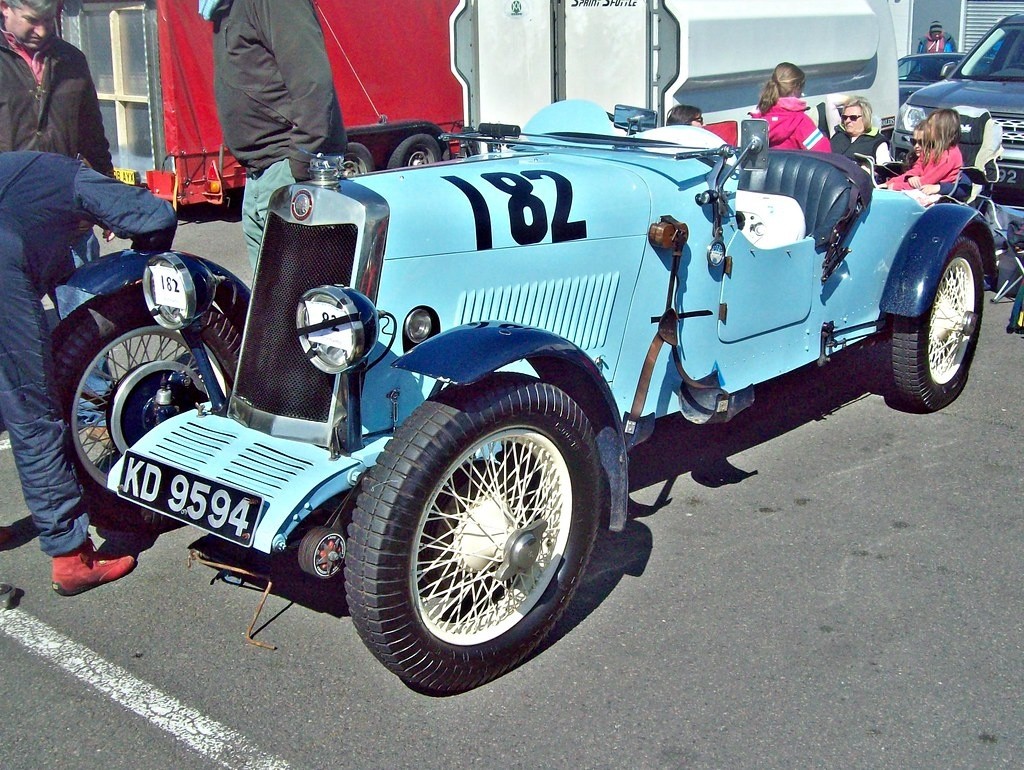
[57,0,899,211]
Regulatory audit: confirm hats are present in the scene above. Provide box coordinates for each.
[930,20,944,31]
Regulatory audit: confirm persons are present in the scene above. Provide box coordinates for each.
[829,94,890,178]
[196,0,348,270]
[876,106,973,208]
[0,0,116,450]
[916,19,958,79]
[746,60,832,154]
[0,150,178,596]
[666,106,707,129]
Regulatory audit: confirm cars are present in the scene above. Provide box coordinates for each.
[42,99,998,697]
[814,53,966,112]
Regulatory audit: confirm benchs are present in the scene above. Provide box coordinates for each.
[736,148,854,235]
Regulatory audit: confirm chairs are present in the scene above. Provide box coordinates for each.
[875,107,1024,300]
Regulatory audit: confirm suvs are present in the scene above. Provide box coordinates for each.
[890,13,1024,189]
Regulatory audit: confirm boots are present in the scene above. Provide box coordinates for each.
[0,526,11,543]
[51,537,135,599]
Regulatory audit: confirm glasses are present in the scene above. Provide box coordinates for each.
[839,114,861,121]
[910,138,928,146]
[695,116,704,124]
